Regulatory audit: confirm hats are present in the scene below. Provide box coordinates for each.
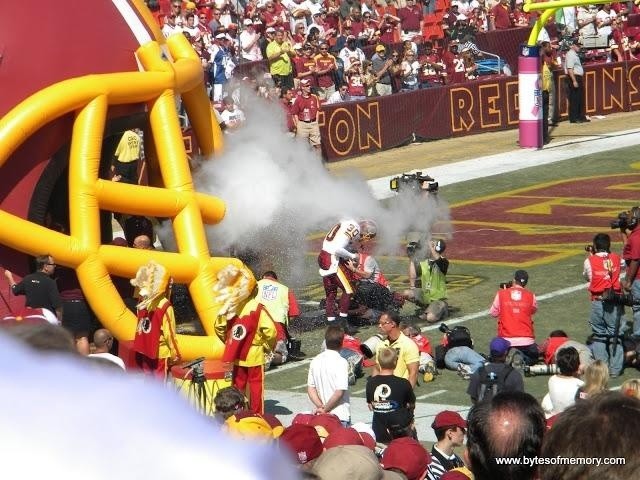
[569,37,584,45]
[490,337,511,354]
[515,270,528,284]
[279,410,474,480]
[375,45,385,52]
[223,411,286,446]
[300,79,311,86]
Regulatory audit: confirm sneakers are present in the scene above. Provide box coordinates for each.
[423,361,433,382]
[348,361,356,385]
[457,363,472,379]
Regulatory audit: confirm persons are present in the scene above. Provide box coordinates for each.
[3,255,65,325]
[106,2,640,185]
[51,265,94,356]
[133,181,638,479]
[86,329,129,372]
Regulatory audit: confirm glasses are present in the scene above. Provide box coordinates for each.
[378,321,392,325]
[48,263,55,266]
[104,337,113,343]
[173,5,221,19]
[320,48,328,50]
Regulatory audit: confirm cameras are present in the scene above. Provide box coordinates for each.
[584,244,592,252]
[500,280,512,288]
[438,322,450,333]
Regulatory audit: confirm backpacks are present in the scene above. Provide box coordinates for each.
[446,326,472,348]
[478,366,514,402]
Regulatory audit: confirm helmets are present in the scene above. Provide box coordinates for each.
[432,240,445,253]
[359,221,376,240]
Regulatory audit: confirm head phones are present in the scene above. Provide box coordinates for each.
[631,207,638,224]
[435,241,441,252]
